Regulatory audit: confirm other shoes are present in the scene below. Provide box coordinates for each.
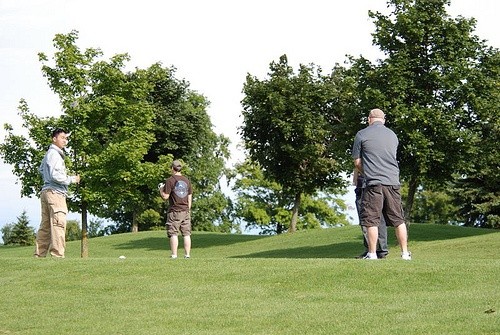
[401,251,411,260]
[363,253,377,259]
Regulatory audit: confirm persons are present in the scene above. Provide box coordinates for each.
[351,108,411,261]
[34,127,80,259]
[157,159,193,260]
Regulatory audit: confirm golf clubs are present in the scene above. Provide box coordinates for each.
[70,101,79,176]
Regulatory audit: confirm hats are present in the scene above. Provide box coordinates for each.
[170,160,183,171]
[369,109,384,119]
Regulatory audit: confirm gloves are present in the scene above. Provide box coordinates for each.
[158,183,165,190]
